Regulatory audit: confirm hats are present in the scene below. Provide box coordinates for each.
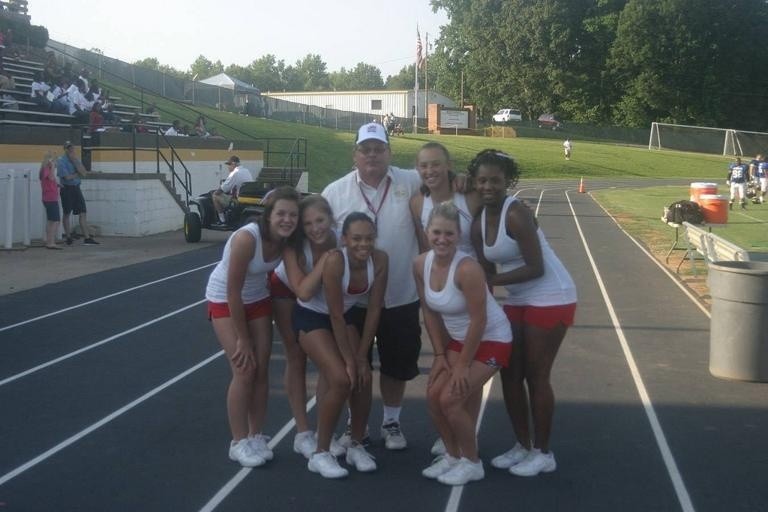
[225,156,240,165]
[64,141,72,149]
[354,121,389,145]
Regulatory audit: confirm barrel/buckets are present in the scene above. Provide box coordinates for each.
[700,194,729,225]
[689,182,718,204]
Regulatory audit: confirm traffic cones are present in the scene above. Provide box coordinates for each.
[578,176,585,193]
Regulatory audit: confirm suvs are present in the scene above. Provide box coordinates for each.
[492,109,521,123]
[537,113,560,131]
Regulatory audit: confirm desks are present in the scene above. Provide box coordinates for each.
[661,217,728,264]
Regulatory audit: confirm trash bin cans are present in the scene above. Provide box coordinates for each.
[706,259,767,382]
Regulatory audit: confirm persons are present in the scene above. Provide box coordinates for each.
[56,140,101,246]
[381,110,405,139]
[0,27,211,146]
[747,152,768,204]
[725,157,750,211]
[410,200,512,487]
[202,183,301,470]
[467,147,579,477]
[268,193,347,461]
[289,211,389,481]
[38,148,65,250]
[211,155,253,227]
[562,138,573,161]
[320,120,471,453]
[406,141,488,458]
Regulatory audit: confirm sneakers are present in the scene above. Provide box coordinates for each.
[430,437,447,454]
[83,238,103,246]
[293,430,319,458]
[210,218,227,227]
[67,238,72,246]
[246,433,274,460]
[437,457,485,485]
[336,417,369,449]
[48,245,62,250]
[315,431,345,455]
[380,418,406,449]
[422,448,463,478]
[345,443,377,472]
[491,436,534,468]
[509,448,556,477]
[228,438,266,467]
[308,451,349,478]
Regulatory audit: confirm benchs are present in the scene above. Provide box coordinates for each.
[677,221,750,274]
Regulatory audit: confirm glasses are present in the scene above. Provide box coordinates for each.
[359,146,385,153]
